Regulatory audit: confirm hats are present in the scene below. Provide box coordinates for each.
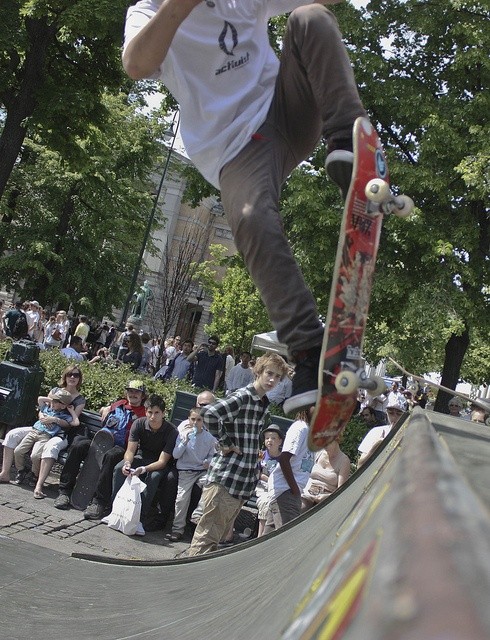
[48,390,72,404]
[260,424,284,441]
[386,395,408,413]
[125,380,147,394]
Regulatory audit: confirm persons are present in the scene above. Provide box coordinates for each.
[470,408,488,424]
[14,390,80,487]
[188,352,289,557]
[255,424,285,538]
[358,392,409,468]
[122,0,366,415]
[143,391,216,532]
[53,380,148,519]
[448,396,462,417]
[353,392,366,414]
[111,394,179,523]
[0,298,255,395]
[362,407,382,428]
[267,355,293,404]
[168,407,217,540]
[300,426,351,515]
[1,366,86,499]
[263,405,315,530]
[388,381,407,409]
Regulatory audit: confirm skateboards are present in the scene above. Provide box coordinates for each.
[307,117,414,453]
[69,428,114,510]
[388,357,490,427]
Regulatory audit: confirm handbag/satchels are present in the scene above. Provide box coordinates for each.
[155,353,181,380]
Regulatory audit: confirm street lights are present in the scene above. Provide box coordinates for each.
[118,109,182,331]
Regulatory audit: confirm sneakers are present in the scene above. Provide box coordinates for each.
[83,496,111,520]
[54,489,70,510]
[169,532,183,542]
[283,345,321,415]
[100,512,112,524]
[135,521,145,536]
[15,468,33,486]
[26,471,38,486]
[325,144,354,202]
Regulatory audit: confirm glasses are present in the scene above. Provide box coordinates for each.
[67,373,80,378]
[53,399,66,405]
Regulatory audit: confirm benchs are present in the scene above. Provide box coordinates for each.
[27,409,105,473]
[156,390,295,539]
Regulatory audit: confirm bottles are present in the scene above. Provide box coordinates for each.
[187,427,199,441]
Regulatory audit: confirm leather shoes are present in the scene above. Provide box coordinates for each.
[34,489,47,498]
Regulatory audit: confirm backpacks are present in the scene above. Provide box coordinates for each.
[122,332,130,347]
[12,313,28,338]
